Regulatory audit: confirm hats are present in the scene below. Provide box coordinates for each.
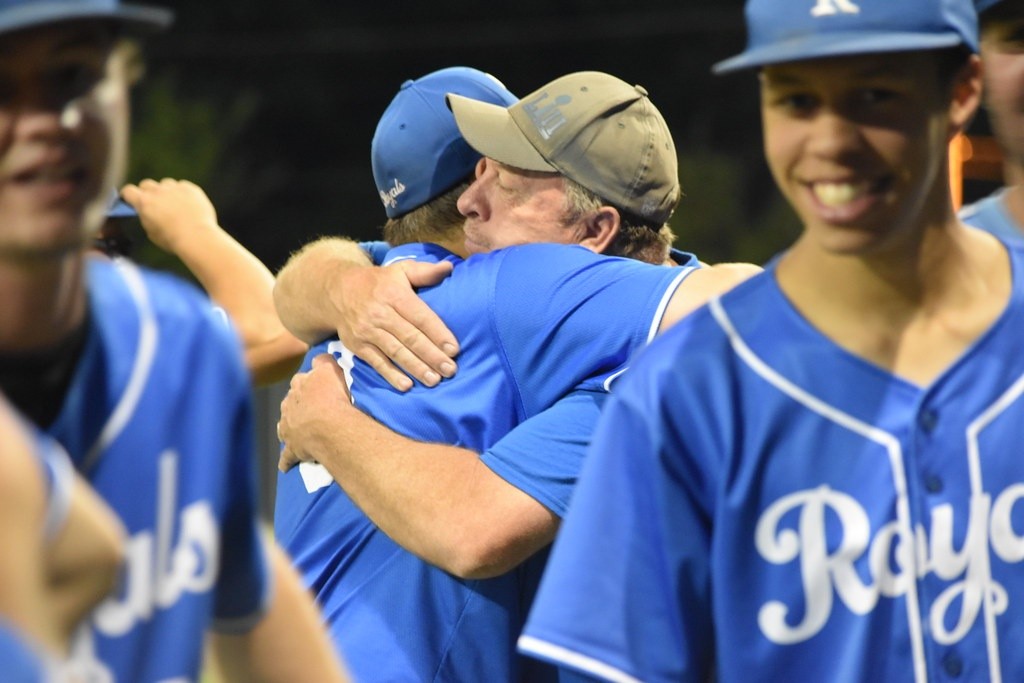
[370,65,523,218]
[0,1,174,39]
[445,70,679,225]
[712,0,981,76]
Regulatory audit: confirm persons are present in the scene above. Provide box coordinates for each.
[93,64,771,683]
[515,0,1024,683]
[1,0,356,683]
